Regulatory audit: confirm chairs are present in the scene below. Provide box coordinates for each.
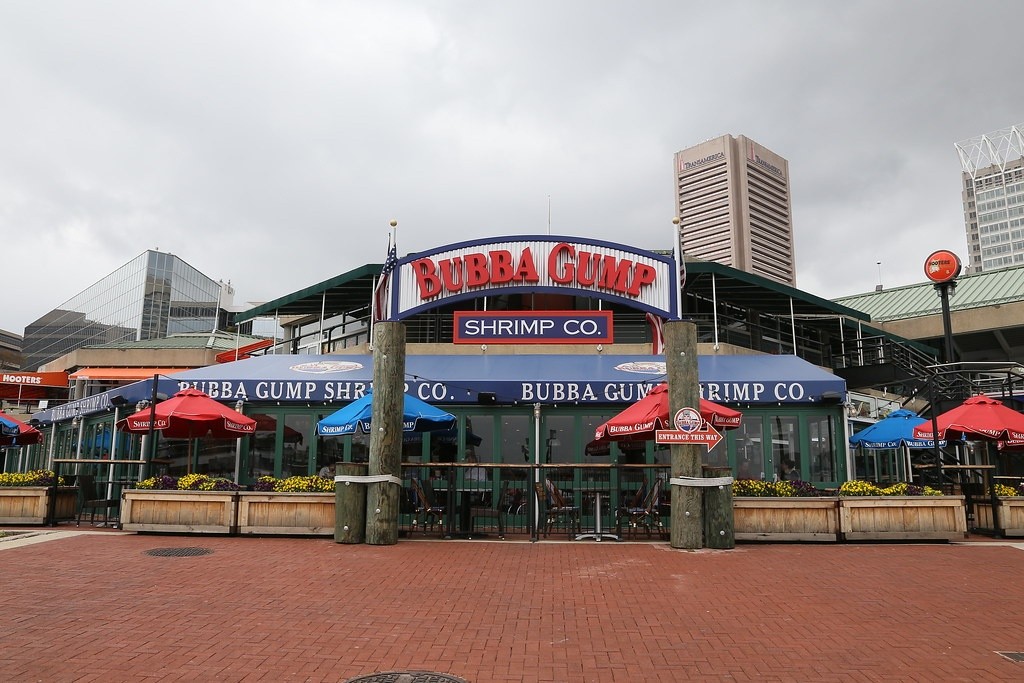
[77,475,120,527]
[534,483,580,540]
[413,476,459,538]
[468,480,509,539]
[545,478,582,536]
[622,478,664,541]
[617,479,648,537]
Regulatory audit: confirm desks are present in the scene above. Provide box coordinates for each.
[572,487,624,537]
[434,488,492,537]
[96,481,141,499]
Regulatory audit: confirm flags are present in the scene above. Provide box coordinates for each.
[373,242,398,320]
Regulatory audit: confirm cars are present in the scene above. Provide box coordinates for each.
[552,505,579,515]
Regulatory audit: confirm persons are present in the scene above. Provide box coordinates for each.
[780,459,800,481]
[319,458,339,479]
[463,447,488,506]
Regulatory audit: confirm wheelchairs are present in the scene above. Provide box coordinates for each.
[506,489,527,515]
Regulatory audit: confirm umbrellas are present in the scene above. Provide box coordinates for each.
[914,394,1024,456]
[199,414,303,451]
[0,409,42,447]
[118,388,256,443]
[73,431,130,458]
[848,408,967,454]
[403,427,483,446]
[585,433,671,456]
[593,378,740,440]
[313,386,459,443]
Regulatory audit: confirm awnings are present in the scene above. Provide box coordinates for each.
[0,371,69,406]
[68,367,194,380]
